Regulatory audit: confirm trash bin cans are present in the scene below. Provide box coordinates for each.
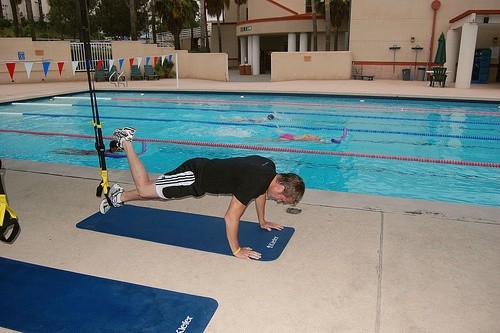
[239,64,245,75]
[402,69,410,81]
[245,64,251,75]
[418,68,425,81]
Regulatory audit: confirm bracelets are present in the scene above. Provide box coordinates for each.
[232,246,241,255]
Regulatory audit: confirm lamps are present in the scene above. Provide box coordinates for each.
[493,37,497,43]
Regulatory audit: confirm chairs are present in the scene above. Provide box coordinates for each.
[130,64,144,81]
[430,66,448,88]
[143,65,160,81]
[94,64,125,82]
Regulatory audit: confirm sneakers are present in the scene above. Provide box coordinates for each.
[112,126,137,150]
[99,183,124,215]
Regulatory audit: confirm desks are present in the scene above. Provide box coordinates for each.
[426,71,452,87]
[239,64,252,75]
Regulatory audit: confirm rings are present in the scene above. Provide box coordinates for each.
[248,254,250,257]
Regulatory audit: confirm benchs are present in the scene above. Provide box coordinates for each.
[353,74,375,81]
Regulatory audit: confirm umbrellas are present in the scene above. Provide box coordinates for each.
[434,32,446,67]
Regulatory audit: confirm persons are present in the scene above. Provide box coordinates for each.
[99,126,306,261]
[47,140,146,158]
[251,127,347,144]
[220,113,286,123]
